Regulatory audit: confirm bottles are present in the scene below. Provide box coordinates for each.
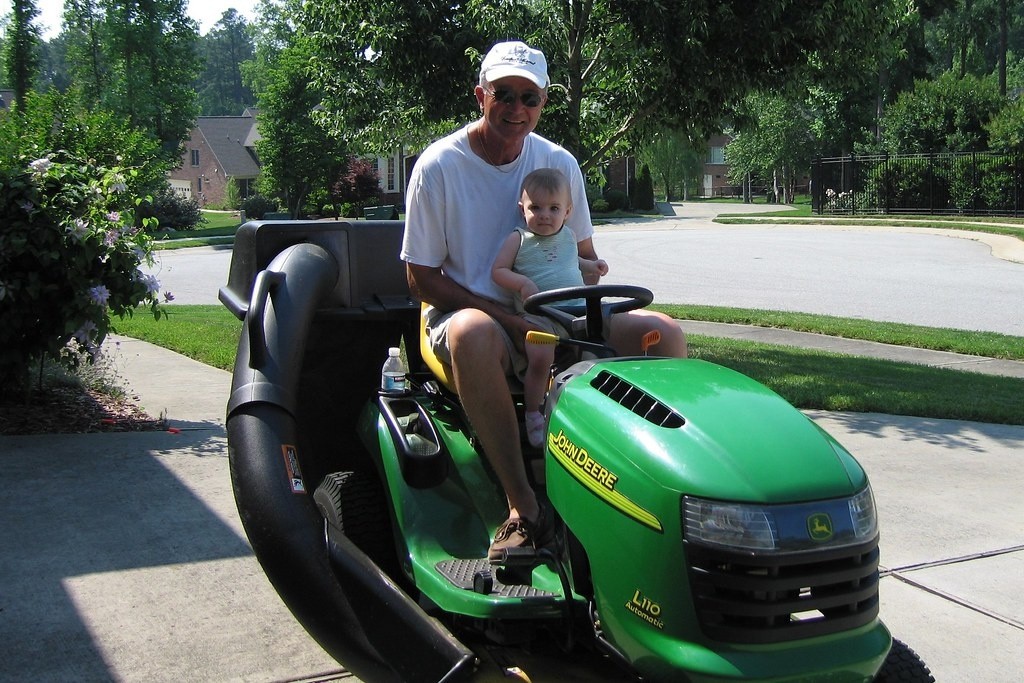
[382,347,405,390]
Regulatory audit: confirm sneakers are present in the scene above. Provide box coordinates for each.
[488,501,545,566]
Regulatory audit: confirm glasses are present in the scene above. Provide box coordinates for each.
[482,84,545,108]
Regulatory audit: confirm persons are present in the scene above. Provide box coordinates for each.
[400,41,688,565]
[492,168,609,449]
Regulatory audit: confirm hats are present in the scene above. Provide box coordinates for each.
[478,41,550,94]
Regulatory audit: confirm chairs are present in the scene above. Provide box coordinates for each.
[417,300,457,396]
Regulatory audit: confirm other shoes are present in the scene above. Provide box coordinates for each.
[525,411,545,448]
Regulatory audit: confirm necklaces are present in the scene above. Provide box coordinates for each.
[477,122,524,173]
